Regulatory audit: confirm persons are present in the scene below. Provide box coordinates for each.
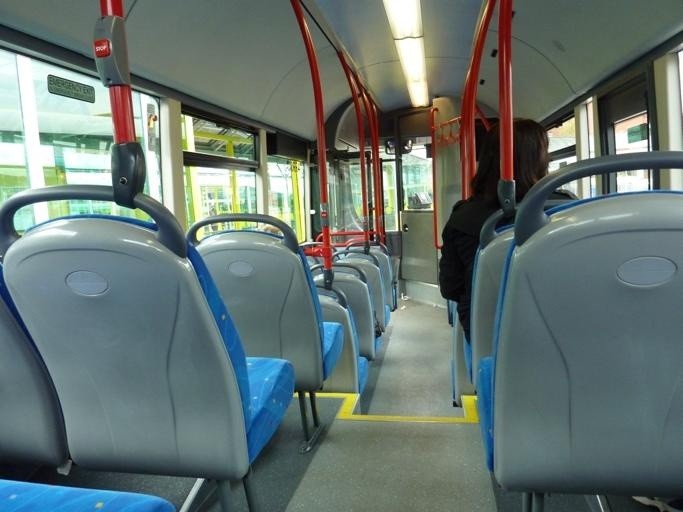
[437,116,579,347]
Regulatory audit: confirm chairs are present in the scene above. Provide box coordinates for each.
[444,151,683,510]
[2,181,401,511]
